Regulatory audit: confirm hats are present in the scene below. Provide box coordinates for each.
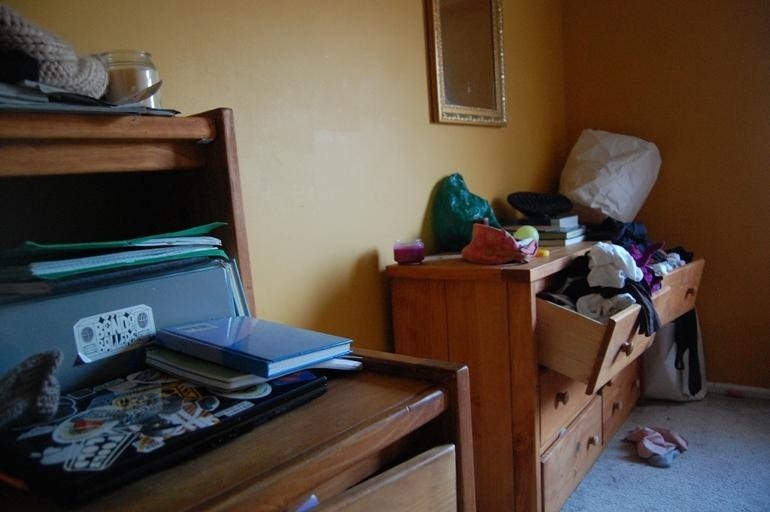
[462,223,539,263]
[1,349,63,428]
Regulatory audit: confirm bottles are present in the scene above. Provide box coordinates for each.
[100,49,162,111]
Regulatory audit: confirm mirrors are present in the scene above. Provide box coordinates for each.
[426,0,508,126]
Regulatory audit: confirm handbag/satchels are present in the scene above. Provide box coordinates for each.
[559,129,662,223]
[642,304,707,401]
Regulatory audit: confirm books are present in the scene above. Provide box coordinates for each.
[145,260,365,391]
[500,224,586,247]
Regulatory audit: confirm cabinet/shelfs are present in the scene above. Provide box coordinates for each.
[0,351,476,511]
[383,238,706,511]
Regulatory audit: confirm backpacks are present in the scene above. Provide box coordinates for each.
[435,173,502,251]
